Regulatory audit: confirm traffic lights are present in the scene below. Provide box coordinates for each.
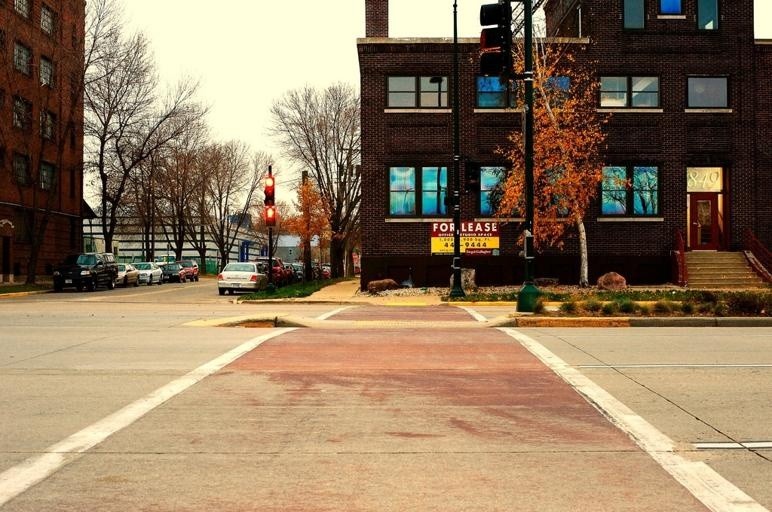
[480,0,516,82]
[463,158,482,190]
[265,176,277,228]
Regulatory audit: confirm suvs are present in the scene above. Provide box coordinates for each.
[53,252,118,292]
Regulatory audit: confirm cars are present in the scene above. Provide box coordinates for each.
[217,258,331,295]
[115,260,200,288]
